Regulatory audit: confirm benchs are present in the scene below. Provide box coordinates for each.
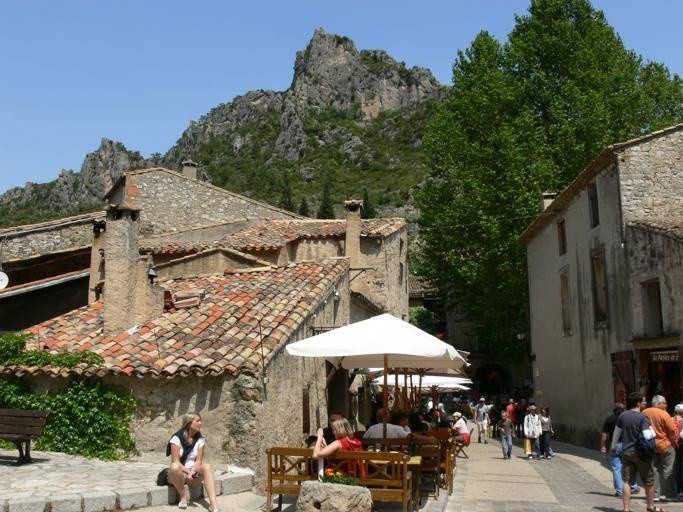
[0,408,51,466]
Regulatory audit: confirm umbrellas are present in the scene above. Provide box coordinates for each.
[285,312,474,454]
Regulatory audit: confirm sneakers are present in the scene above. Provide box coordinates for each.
[654,494,682,502]
[178,495,189,508]
[208,505,220,512]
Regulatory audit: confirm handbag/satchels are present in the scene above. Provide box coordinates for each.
[524,438,532,454]
[637,432,655,460]
[157,468,169,486]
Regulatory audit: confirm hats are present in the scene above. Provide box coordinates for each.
[452,412,461,418]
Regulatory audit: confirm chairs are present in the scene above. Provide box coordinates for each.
[265,399,473,511]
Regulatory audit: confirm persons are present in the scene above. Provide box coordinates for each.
[600,394,683,512]
[308,395,556,479]
[166,412,223,512]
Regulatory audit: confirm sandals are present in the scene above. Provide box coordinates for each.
[647,507,664,512]
